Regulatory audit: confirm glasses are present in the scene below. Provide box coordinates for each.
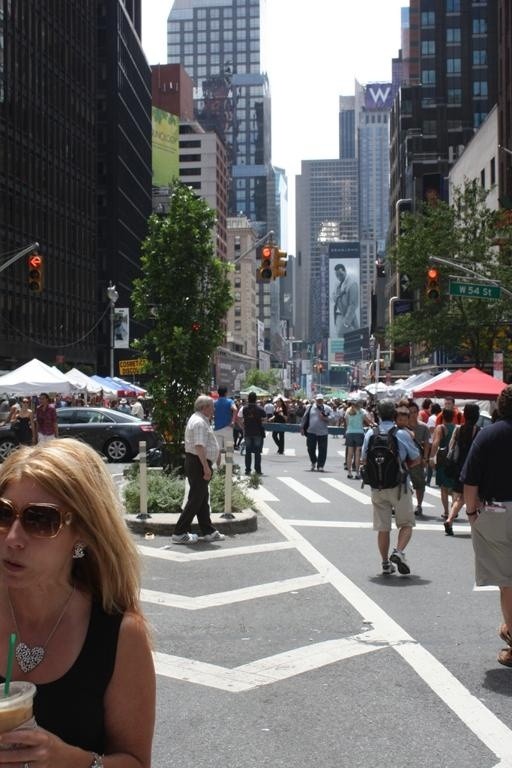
[1,498,75,539]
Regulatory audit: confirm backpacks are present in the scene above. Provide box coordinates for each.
[361,426,405,489]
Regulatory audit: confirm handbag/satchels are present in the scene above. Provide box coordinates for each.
[304,419,309,430]
[436,446,450,465]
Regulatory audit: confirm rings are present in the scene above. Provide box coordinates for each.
[24,763,29,768]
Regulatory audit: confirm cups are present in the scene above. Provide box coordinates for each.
[1,682,37,743]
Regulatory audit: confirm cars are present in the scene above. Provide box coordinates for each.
[1,406,161,461]
[462,408,495,430]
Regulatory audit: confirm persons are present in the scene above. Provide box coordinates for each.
[171,395,227,545]
[360,399,422,577]
[0,393,150,444]
[214,385,482,534]
[491,408,501,422]
[331,263,358,336]
[0,437,156,768]
[458,384,511,667]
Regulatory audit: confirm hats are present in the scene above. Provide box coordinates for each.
[315,394,323,400]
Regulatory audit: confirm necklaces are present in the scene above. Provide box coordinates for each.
[5,584,74,674]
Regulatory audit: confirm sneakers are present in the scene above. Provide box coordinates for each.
[498,623,512,666]
[172,530,227,544]
[381,547,411,573]
[309,463,323,472]
[442,510,459,534]
[245,471,263,474]
[415,504,423,516]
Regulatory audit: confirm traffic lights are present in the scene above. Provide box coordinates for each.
[274,248,287,279]
[426,267,440,300]
[316,364,323,374]
[256,246,274,284]
[27,252,45,295]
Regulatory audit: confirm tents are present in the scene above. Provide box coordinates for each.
[0,359,150,408]
[323,368,510,404]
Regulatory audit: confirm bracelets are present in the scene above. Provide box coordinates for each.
[89,751,103,768]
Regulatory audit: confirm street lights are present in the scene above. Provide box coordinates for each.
[106,279,119,376]
